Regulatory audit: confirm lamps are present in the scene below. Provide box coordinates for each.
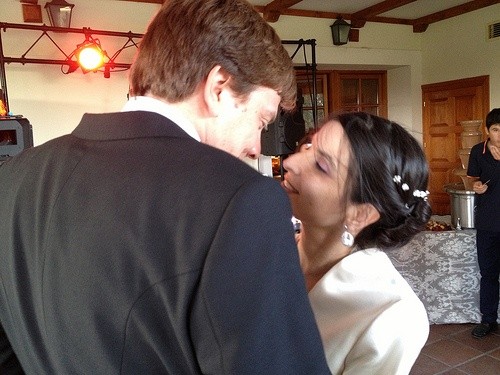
[329,16,353,45]
[45,0,75,33]
[74,41,107,75]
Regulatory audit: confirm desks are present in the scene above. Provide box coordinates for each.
[291,213,500,325]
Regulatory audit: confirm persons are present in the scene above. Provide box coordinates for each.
[466,108,500,340]
[280,111,431,375]
[0,1,334,374]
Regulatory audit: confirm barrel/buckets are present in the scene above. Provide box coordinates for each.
[444,182,475,229]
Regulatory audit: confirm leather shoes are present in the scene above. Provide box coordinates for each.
[470,322,498,339]
